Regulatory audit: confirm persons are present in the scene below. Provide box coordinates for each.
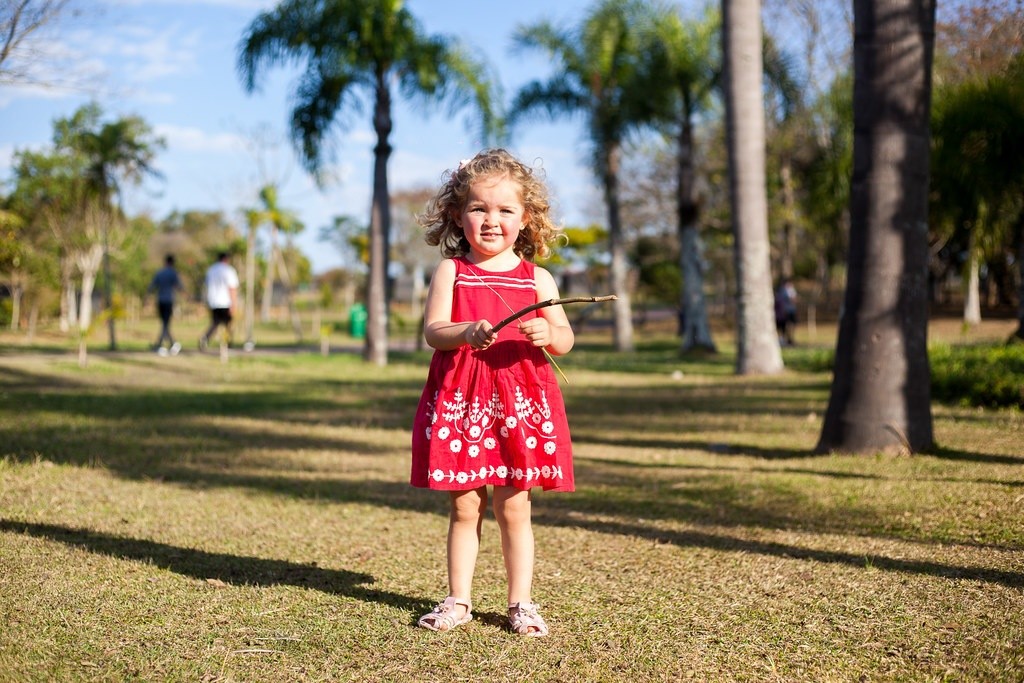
[197,252,239,353]
[146,254,186,357]
[408,149,577,639]
[774,277,799,347]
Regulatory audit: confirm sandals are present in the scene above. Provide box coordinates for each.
[418,596,473,633]
[507,602,548,637]
